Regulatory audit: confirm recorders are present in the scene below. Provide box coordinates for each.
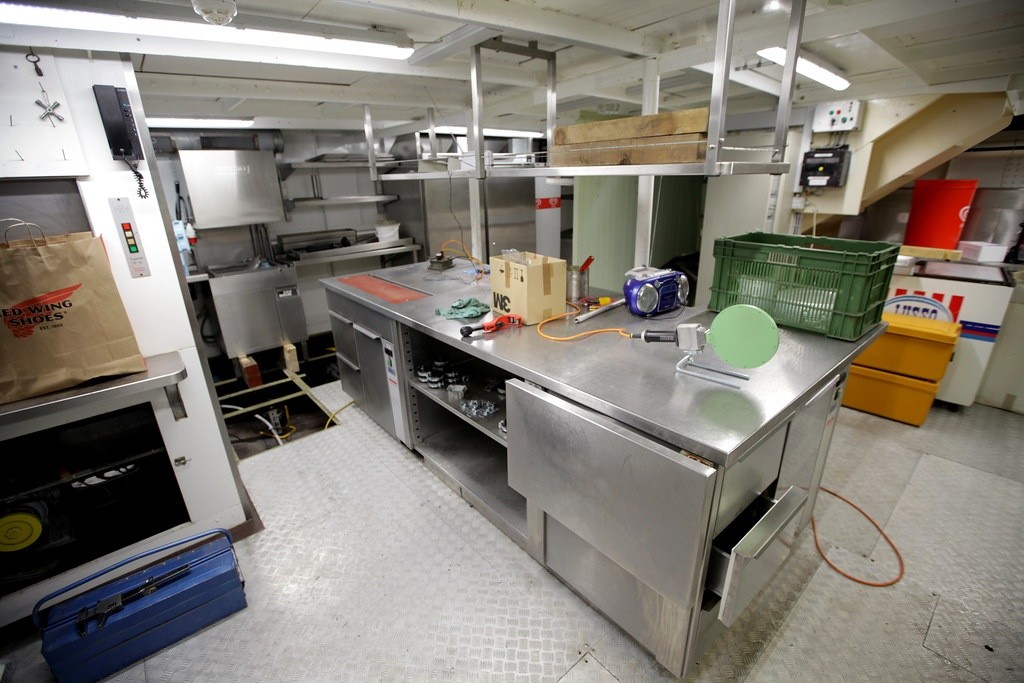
[623,267,689,317]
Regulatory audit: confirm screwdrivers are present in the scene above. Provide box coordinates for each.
[580,256,594,271]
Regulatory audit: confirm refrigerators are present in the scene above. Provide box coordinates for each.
[886,257,1017,409]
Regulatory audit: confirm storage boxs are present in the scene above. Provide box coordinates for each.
[840,364,939,428]
[706,229,902,341]
[489,251,568,326]
[851,313,965,380]
[957,239,1009,264]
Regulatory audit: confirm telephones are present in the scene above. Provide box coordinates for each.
[92,83,145,162]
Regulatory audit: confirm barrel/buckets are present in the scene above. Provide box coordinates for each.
[904,178,979,250]
[374,223,400,242]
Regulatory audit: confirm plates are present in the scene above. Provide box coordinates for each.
[736,273,837,328]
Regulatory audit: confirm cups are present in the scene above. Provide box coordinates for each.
[566,266,589,303]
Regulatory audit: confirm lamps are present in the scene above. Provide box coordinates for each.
[757,45,852,92]
[0,0,414,62]
